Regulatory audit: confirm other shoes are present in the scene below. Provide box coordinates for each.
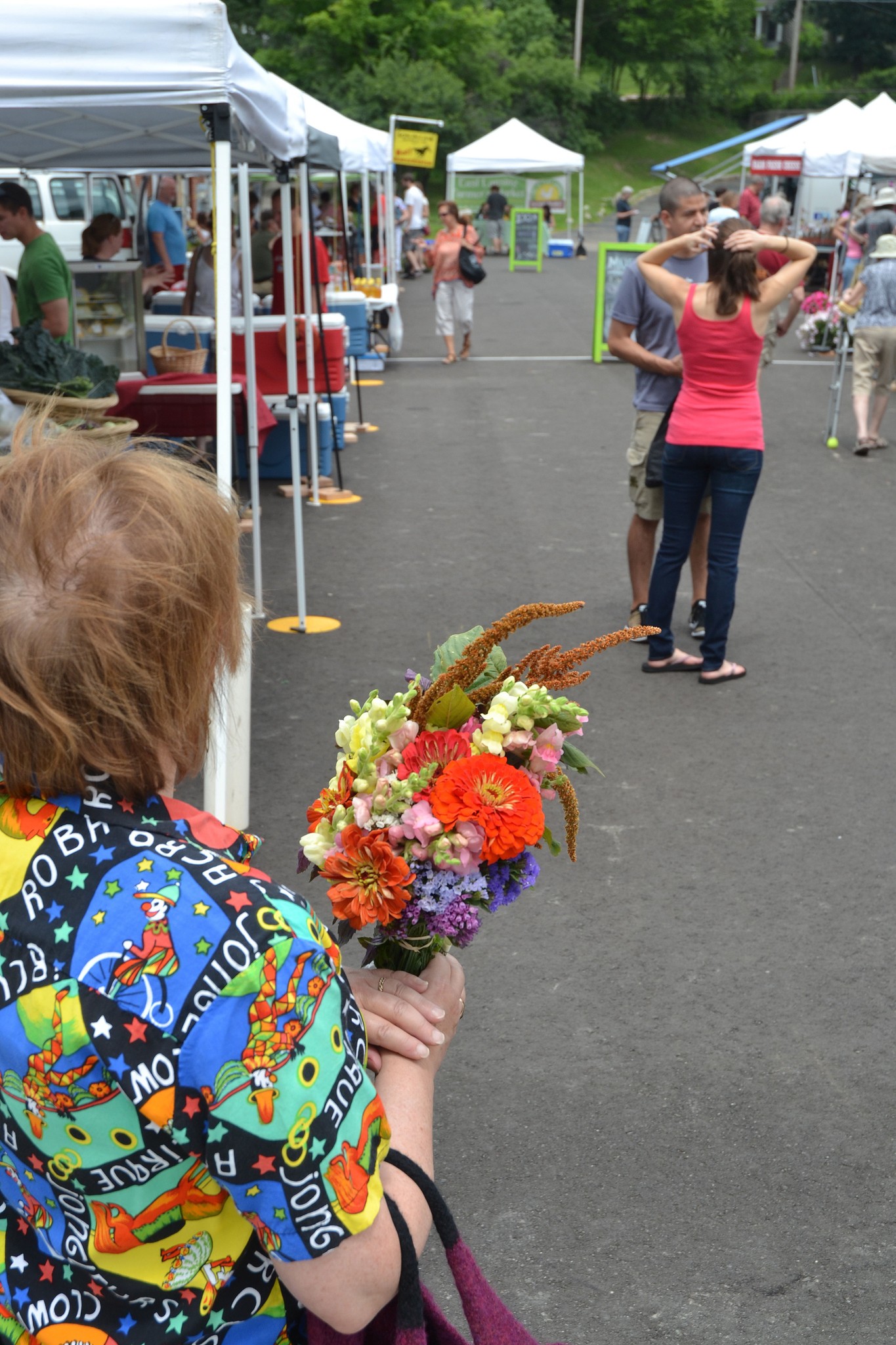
[442,341,472,364]
[402,267,424,282]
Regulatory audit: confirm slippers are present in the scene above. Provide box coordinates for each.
[641,650,703,674]
[699,658,746,685]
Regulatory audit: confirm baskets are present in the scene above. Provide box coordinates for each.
[0,385,120,419]
[55,414,139,450]
[149,319,209,375]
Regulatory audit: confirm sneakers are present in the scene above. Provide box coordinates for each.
[623,602,647,644]
[688,598,706,638]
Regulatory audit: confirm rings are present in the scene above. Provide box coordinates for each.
[377,976,387,992]
[459,998,466,1019]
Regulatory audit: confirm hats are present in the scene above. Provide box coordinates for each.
[868,233,896,259]
[872,187,896,207]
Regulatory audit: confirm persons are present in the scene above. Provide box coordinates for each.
[483,184,508,254]
[541,204,556,231]
[840,234,895,457]
[607,176,711,646]
[433,200,485,366]
[0,383,473,1345]
[634,216,821,685]
[413,180,431,261]
[754,193,804,379]
[402,172,425,276]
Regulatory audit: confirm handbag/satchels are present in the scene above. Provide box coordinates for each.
[457,246,486,285]
[287,1144,544,1345]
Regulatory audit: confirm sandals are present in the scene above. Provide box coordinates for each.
[852,434,890,457]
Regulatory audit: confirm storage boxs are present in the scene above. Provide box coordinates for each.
[94,292,386,483]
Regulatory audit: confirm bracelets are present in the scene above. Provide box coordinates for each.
[779,234,790,255]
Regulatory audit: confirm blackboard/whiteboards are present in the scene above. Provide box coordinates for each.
[595,242,659,352]
[510,207,544,262]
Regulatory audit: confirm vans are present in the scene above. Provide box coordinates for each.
[0,168,135,291]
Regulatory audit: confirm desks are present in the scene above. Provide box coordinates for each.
[347,281,404,370]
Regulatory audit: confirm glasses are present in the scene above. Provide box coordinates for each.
[438,212,450,217]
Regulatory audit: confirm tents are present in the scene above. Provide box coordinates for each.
[710,87,895,333]
[0,0,401,635]
[445,117,587,250]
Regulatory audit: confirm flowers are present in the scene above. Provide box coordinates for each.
[295,601,670,973]
